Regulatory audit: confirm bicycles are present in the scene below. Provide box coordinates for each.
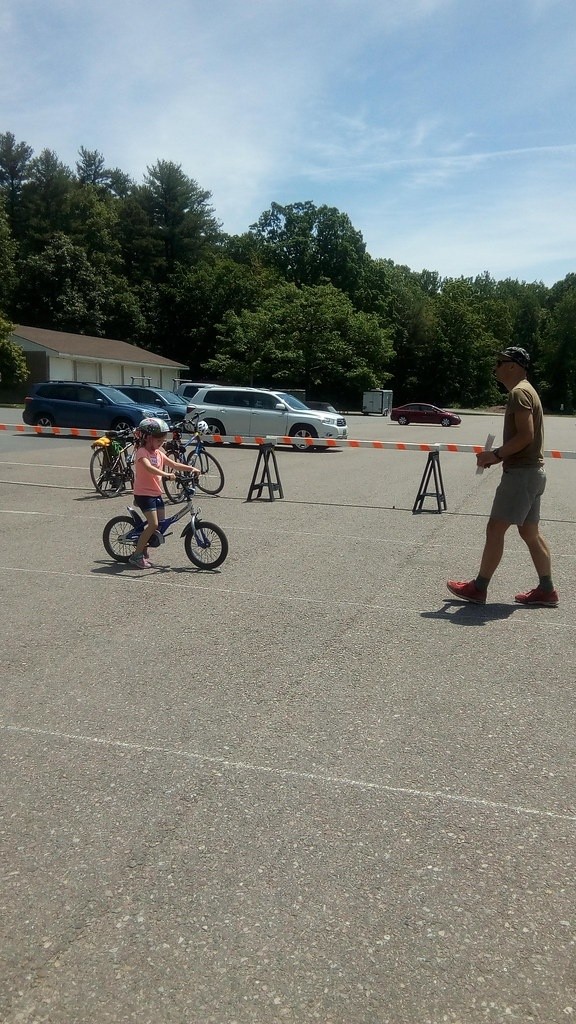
[102,472,229,569]
[89,410,225,503]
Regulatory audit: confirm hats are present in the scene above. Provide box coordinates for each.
[498,345,530,369]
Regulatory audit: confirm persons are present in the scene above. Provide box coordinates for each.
[128,417,200,569]
[446,347,559,606]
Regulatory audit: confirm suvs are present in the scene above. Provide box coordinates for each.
[22,382,349,452]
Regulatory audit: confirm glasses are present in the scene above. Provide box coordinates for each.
[498,359,515,366]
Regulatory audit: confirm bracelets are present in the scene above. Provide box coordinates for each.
[493,448,502,460]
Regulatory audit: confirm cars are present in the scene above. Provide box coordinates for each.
[390,403,462,427]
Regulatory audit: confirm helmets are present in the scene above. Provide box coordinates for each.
[139,418,170,434]
[197,421,208,435]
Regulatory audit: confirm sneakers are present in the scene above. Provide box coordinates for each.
[446,580,488,605]
[515,586,559,606]
[129,554,151,569]
